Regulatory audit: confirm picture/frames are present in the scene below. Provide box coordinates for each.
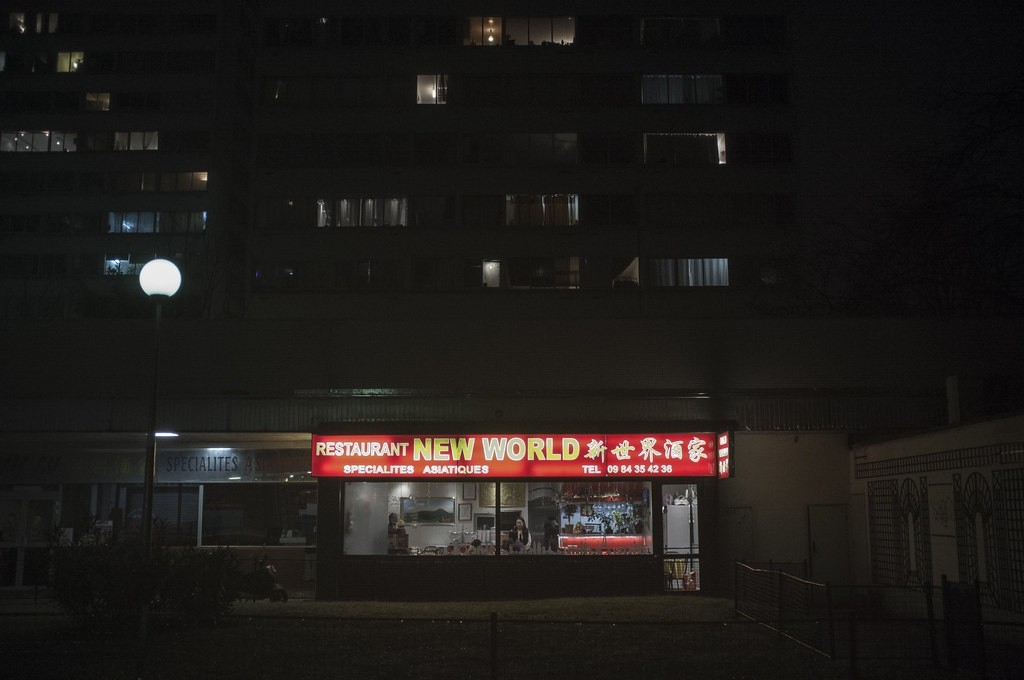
[399,497,456,526]
[458,503,473,522]
[462,481,476,500]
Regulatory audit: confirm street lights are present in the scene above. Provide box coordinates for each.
[137,258,184,609]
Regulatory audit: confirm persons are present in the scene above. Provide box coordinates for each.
[513,518,532,550]
[388,513,400,553]
[543,515,560,551]
[394,520,409,550]
[500,526,511,551]
[507,531,528,554]
